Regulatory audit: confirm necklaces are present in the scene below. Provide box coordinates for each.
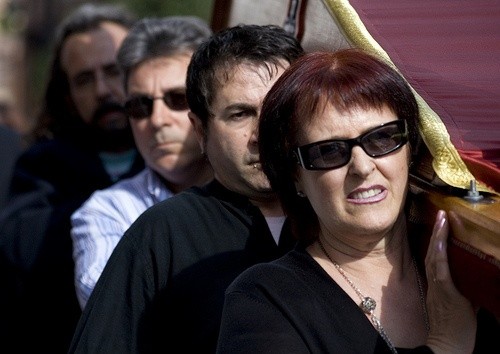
[318,237,430,354]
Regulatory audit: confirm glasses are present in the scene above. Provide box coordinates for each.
[124,87,191,119]
[290,115,413,172]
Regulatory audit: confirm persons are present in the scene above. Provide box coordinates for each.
[0,1,149,354]
[68,16,214,316]
[217,51,500,354]
[68,25,307,354]
